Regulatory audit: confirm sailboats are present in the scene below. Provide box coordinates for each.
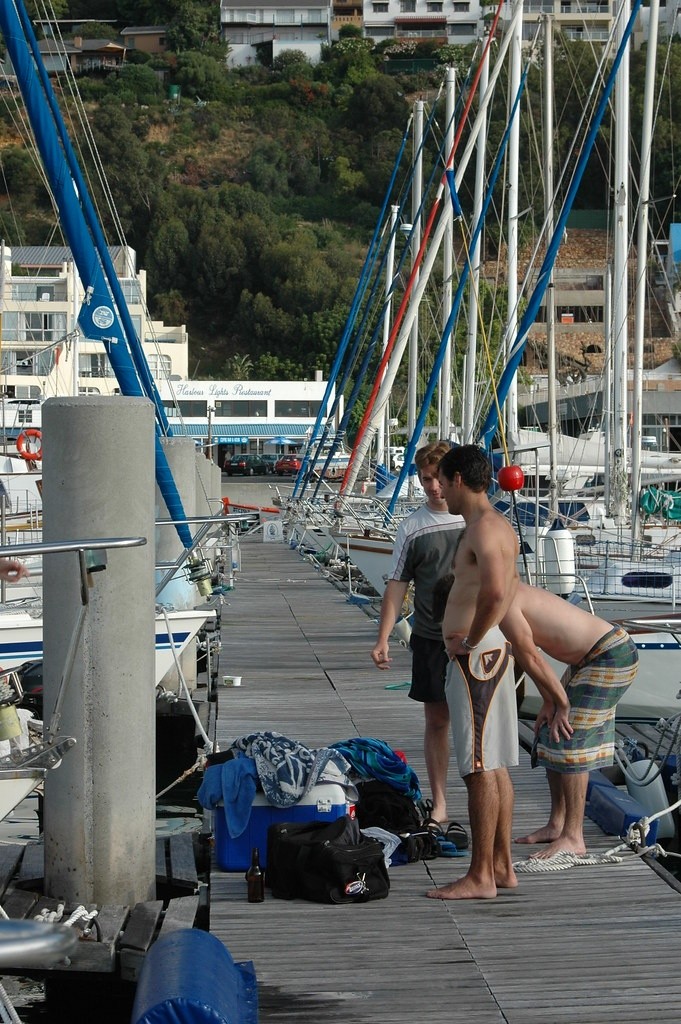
[266,1,681,883]
[0,0,240,1024]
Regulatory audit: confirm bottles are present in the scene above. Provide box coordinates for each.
[247,848,265,903]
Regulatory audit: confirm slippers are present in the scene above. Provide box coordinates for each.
[421,818,444,838]
[445,821,468,849]
[436,836,469,857]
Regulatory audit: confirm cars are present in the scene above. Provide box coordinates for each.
[223,454,270,477]
[275,454,303,476]
[260,453,284,475]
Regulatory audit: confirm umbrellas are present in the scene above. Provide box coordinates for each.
[264,436,298,454]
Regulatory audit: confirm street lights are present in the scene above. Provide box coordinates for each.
[207,405,216,459]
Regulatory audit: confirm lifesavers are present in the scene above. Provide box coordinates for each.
[16,430,44,459]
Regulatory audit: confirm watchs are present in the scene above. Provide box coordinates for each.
[462,636,479,651]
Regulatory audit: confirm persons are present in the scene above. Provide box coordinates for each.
[451,558,640,859]
[370,442,467,827]
[426,445,520,899]
[225,451,232,460]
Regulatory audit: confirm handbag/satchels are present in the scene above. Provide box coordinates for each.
[314,839,390,904]
[266,813,362,901]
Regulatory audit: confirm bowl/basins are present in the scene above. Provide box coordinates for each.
[222,676,242,686]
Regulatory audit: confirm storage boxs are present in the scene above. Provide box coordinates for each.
[213,783,346,871]
